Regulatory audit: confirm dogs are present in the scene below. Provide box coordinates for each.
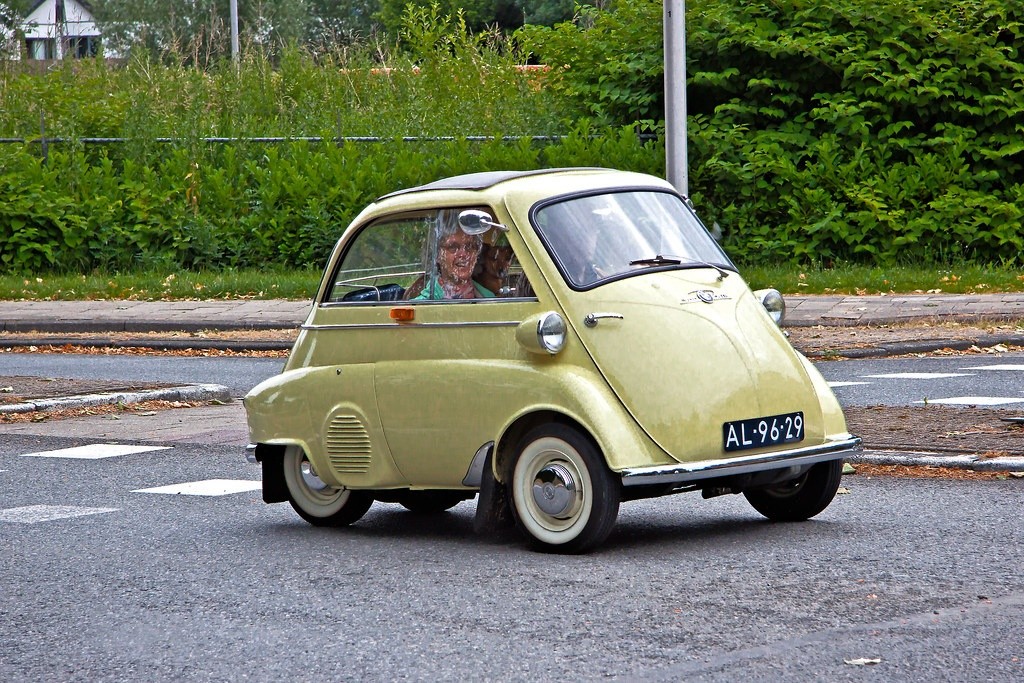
[403,242,514,300]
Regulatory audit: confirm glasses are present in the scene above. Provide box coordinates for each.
[488,253,512,262]
[439,242,479,254]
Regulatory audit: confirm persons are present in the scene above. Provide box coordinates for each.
[515,209,611,297]
[410,208,514,300]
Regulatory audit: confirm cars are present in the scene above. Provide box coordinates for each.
[239,166,864,555]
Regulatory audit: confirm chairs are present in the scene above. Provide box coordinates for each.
[343,282,404,301]
